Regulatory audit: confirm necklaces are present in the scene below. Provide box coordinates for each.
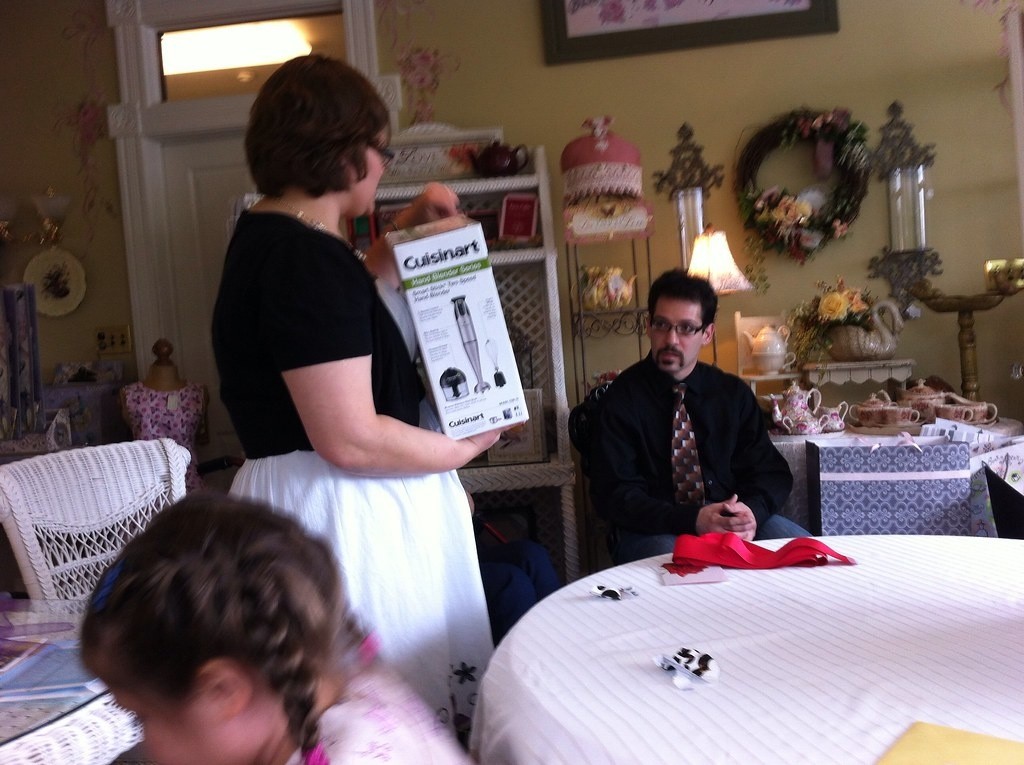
[270,195,368,260]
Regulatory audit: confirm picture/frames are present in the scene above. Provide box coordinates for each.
[543,0,839,67]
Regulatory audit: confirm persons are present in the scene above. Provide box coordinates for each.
[119,337,211,498]
[211,52,498,752]
[76,496,477,765]
[584,268,814,563]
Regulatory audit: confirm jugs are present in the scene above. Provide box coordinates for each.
[819,401,848,431]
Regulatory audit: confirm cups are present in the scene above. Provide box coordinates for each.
[753,352,796,375]
[937,401,998,426]
[884,406,918,425]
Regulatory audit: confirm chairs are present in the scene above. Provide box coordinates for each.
[0,438,247,619]
[735,310,802,401]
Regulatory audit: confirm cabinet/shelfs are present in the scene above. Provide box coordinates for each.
[374,144,578,584]
[568,224,653,405]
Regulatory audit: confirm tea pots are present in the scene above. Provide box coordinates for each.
[743,324,791,355]
[849,391,892,426]
[879,378,947,418]
[769,381,829,436]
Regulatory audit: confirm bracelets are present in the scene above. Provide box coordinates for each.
[391,219,401,231]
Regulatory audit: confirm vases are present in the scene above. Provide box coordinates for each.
[820,301,905,361]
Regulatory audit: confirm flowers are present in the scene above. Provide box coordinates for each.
[781,274,880,390]
[729,103,873,266]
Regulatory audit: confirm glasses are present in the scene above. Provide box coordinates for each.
[367,141,396,169]
[650,317,704,336]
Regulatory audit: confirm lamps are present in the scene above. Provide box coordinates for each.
[681,223,752,369]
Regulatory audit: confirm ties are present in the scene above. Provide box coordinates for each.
[671,383,706,506]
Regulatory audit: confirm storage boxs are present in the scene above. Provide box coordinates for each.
[385,214,529,439]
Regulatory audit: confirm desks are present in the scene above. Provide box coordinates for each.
[0,596,144,765]
[767,418,1024,534]
[798,358,915,409]
[470,534,1023,765]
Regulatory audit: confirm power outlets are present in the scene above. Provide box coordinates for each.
[94,324,131,356]
